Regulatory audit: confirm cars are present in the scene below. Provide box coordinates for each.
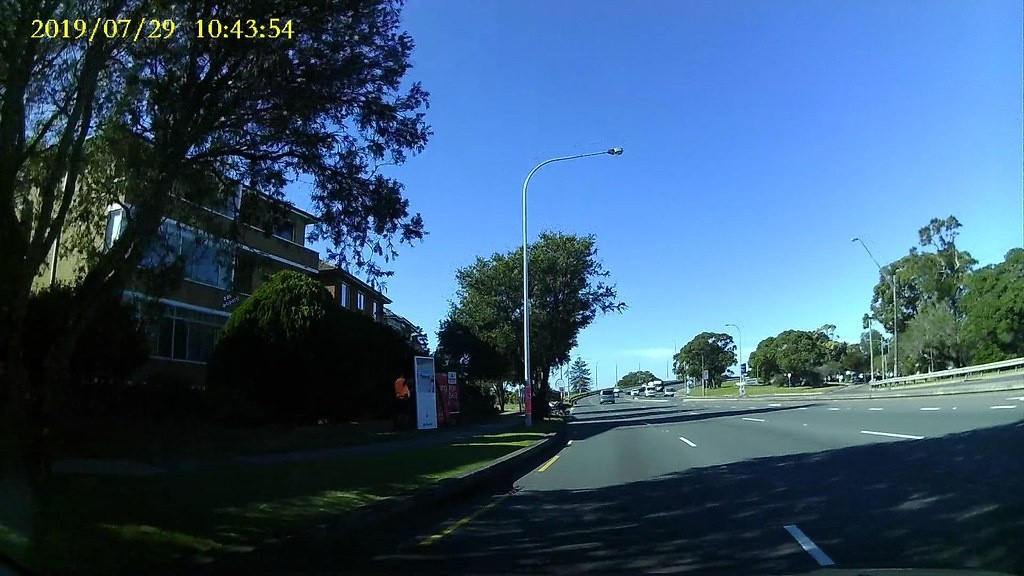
[631,389,640,396]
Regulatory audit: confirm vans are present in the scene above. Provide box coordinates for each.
[663,387,675,397]
[645,386,656,397]
[599,390,615,404]
[640,380,664,393]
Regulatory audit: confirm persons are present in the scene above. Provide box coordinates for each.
[394,372,411,430]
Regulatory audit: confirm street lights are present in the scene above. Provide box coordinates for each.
[673,338,677,380]
[726,324,744,395]
[851,237,897,379]
[692,350,705,396]
[521,146,625,427]
